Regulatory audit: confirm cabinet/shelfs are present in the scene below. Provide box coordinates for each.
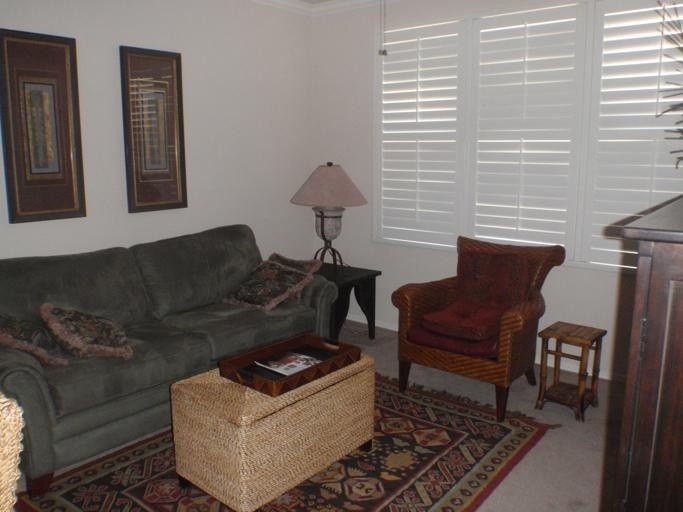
[622,197,683,511]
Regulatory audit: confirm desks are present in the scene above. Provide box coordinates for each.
[534,321,608,422]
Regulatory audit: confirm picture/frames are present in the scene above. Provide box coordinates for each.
[1,28,87,225]
[119,45,188,214]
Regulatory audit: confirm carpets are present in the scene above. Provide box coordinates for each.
[12,371,563,512]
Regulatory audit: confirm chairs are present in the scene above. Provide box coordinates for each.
[391,236,566,422]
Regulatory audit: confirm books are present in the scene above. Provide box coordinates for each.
[254,350,321,376]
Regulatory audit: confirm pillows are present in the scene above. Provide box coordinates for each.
[221,260,314,312]
[39,303,134,359]
[0,312,69,366]
[268,252,322,274]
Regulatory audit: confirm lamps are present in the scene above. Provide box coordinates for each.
[288,162,369,270]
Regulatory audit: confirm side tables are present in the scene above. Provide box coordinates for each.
[313,264,382,340]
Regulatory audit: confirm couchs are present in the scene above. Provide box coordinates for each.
[0,224,338,501]
